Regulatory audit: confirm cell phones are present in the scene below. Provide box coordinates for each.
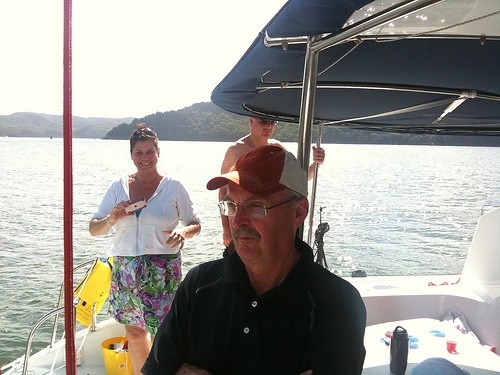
[125,198,147,215]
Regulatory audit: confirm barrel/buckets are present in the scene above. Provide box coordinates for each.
[101,337,135,375]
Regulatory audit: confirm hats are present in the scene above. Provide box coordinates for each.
[206,145,307,198]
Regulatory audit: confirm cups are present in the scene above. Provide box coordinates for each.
[446,340,457,353]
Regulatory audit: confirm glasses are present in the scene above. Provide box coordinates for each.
[253,117,279,126]
[217,195,295,218]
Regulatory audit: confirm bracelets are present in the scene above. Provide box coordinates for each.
[107,215,113,226]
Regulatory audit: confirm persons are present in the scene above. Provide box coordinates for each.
[219,117,325,258]
[89,123,202,375]
[141,144,367,375]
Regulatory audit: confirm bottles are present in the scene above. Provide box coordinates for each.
[390,326,408,375]
[385,330,393,343]
